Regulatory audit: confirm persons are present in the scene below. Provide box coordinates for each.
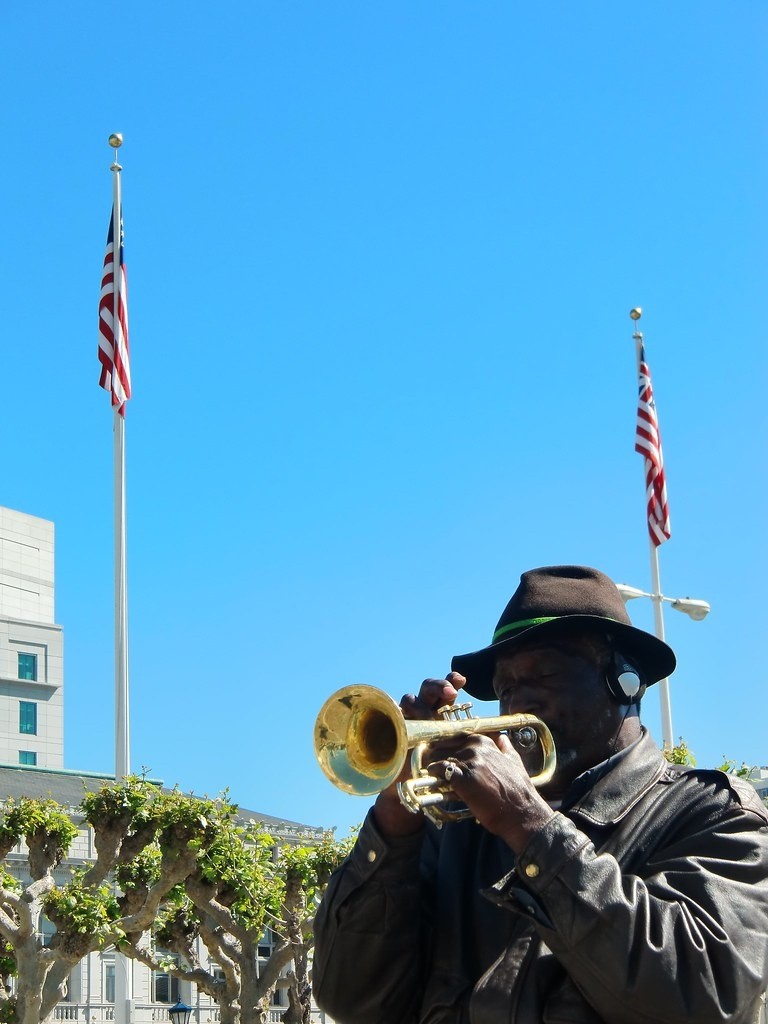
[313,564,768,1024]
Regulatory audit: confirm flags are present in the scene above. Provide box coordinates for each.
[634,342,671,546]
[98,199,131,418]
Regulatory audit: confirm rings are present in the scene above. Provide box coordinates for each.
[444,762,457,781]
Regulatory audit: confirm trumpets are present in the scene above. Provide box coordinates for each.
[312,684,559,825]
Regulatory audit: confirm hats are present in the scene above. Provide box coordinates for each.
[450,563,677,688]
